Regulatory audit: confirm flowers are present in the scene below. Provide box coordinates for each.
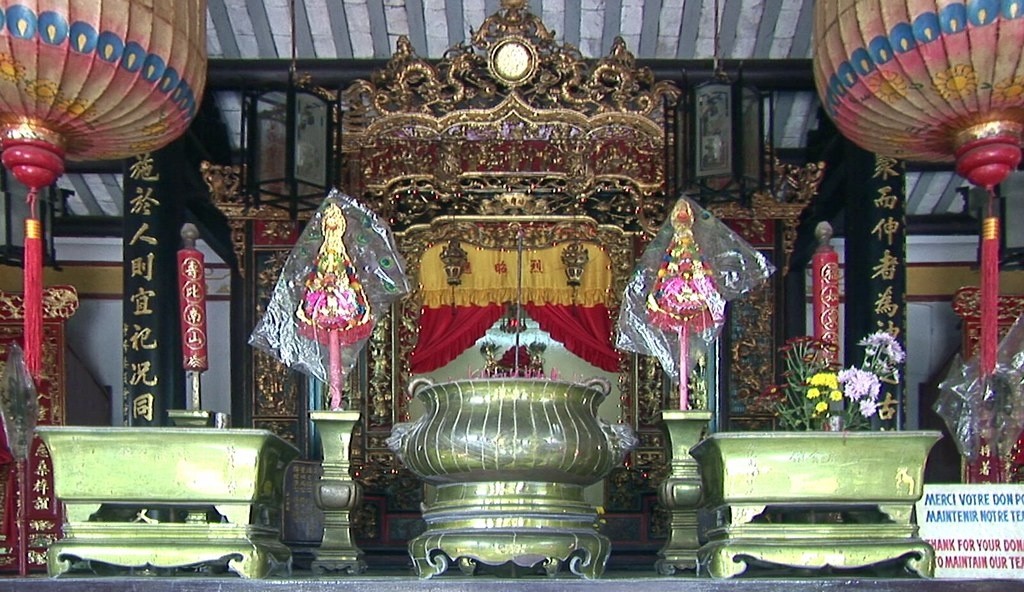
[751,330,905,431]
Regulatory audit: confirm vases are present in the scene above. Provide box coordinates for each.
[34,425,302,582]
[687,429,946,578]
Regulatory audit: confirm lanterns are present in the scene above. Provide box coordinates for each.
[0,0,207,377]
[813,1,1024,381]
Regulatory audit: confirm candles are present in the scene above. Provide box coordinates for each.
[177,247,209,411]
[812,245,839,366]
